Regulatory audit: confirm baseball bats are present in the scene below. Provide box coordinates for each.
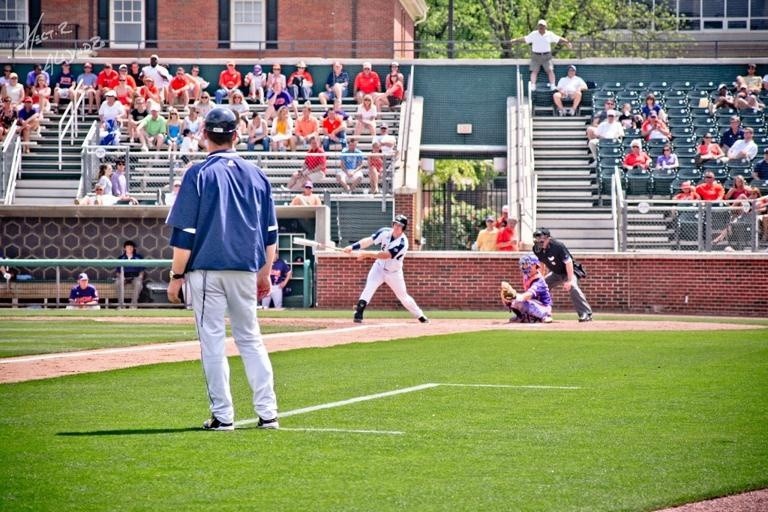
[293,236,355,255]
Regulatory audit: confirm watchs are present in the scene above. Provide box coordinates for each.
[169,269,185,281]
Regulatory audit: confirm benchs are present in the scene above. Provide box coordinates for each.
[0,279,134,309]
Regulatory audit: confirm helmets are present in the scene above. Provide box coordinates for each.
[392,214,407,230]
[519,255,542,274]
[533,228,550,254]
[204,107,238,134]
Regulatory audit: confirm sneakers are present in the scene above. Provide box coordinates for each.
[354,312,363,321]
[420,316,429,323]
[280,184,290,191]
[531,83,558,92]
[256,416,280,430]
[201,414,234,430]
[578,313,593,321]
[510,317,554,323]
[140,145,148,151]
[558,108,575,116]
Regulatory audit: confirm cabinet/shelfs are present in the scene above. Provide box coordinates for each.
[275,233,307,280]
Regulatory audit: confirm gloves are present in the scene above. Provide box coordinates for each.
[4,272,11,280]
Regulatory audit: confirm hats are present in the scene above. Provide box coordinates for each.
[486,205,508,222]
[9,54,161,112]
[538,19,546,27]
[718,63,756,90]
[680,182,690,190]
[649,109,657,117]
[568,65,576,70]
[304,181,314,188]
[225,60,399,76]
[380,123,388,129]
[79,273,89,280]
[607,109,616,117]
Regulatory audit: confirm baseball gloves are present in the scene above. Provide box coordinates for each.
[500,281,517,301]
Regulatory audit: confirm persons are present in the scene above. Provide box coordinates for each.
[261,250,291,309]
[505,17,570,90]
[0,258,21,307]
[496,217,519,251]
[476,214,502,252]
[66,272,101,311]
[502,253,558,327]
[116,239,145,312]
[495,204,509,229]
[341,212,433,325]
[529,226,596,322]
[584,61,768,252]
[1,55,404,207]
[164,106,283,430]
[553,62,590,120]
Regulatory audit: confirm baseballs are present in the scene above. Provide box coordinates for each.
[3,273,11,279]
